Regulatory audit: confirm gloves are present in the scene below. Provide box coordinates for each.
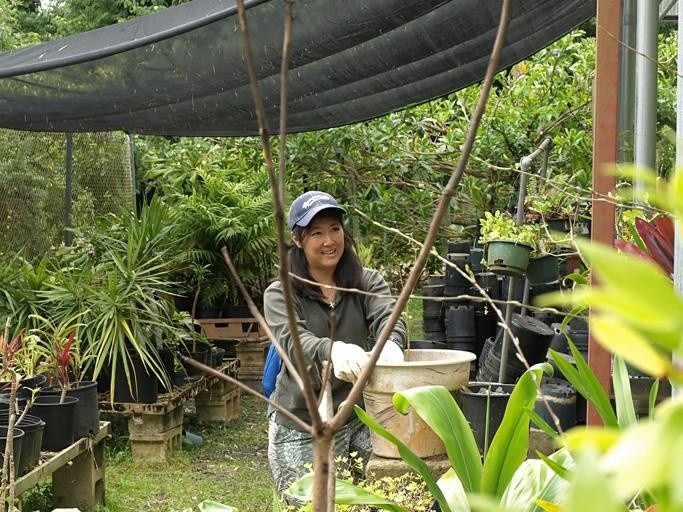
[376,339,404,365]
[331,341,368,384]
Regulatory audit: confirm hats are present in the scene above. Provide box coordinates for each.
[288,190,346,230]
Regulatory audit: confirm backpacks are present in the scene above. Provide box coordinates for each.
[262,332,282,398]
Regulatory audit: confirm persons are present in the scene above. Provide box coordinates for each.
[261,190,409,508]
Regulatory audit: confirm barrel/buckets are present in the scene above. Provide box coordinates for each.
[359,347,475,459]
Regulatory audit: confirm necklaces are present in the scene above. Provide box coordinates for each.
[320,285,336,308]
